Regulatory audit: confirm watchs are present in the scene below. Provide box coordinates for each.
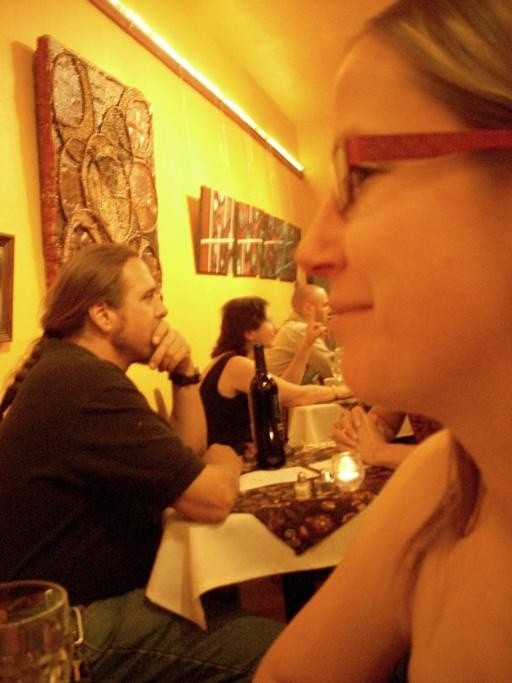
[167,372,200,387]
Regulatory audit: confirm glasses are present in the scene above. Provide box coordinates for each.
[323,126,511,212]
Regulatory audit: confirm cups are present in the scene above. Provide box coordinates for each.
[0,580,69,683]
[331,449,367,492]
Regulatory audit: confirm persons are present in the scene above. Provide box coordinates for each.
[253,0,512,683]
[1,242,290,683]
[199,283,339,465]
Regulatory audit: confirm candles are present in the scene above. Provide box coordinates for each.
[336,452,361,484]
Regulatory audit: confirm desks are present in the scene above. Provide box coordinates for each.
[282,399,405,441]
[144,434,413,631]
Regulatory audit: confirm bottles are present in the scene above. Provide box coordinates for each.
[294,470,334,500]
[246,343,288,467]
